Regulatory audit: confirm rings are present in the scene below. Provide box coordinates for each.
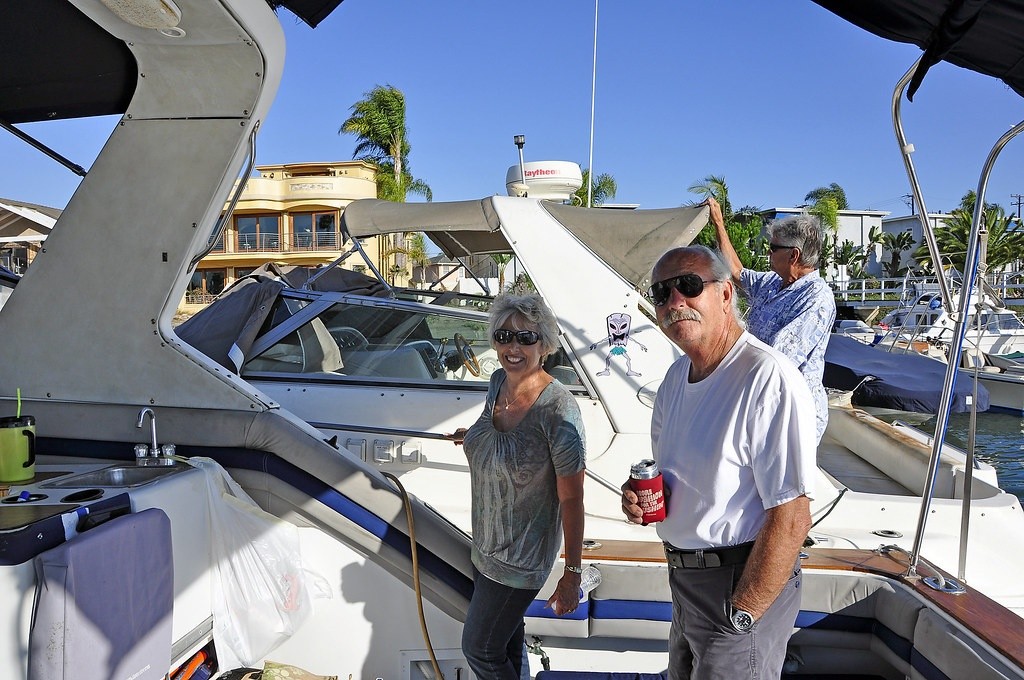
[568,609,575,614]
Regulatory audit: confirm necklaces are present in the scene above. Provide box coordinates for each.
[504,360,542,411]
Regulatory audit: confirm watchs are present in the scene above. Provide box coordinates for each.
[730,606,757,632]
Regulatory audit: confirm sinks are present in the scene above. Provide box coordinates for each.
[38,464,182,489]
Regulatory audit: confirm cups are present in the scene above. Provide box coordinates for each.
[0,415,36,482]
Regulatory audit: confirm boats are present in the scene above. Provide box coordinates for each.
[1,0,1024,680]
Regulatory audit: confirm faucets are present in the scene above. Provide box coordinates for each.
[135,406,161,459]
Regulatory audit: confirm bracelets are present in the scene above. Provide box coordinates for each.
[564,565,584,574]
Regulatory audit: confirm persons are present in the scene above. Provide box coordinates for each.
[697,192,837,556]
[618,244,821,680]
[448,292,590,680]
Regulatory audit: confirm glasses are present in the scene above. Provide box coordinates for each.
[491,329,549,347]
[769,241,799,254]
[645,274,727,307]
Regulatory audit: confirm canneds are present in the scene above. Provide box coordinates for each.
[631,459,659,480]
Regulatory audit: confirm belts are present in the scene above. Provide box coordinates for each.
[660,542,756,572]
